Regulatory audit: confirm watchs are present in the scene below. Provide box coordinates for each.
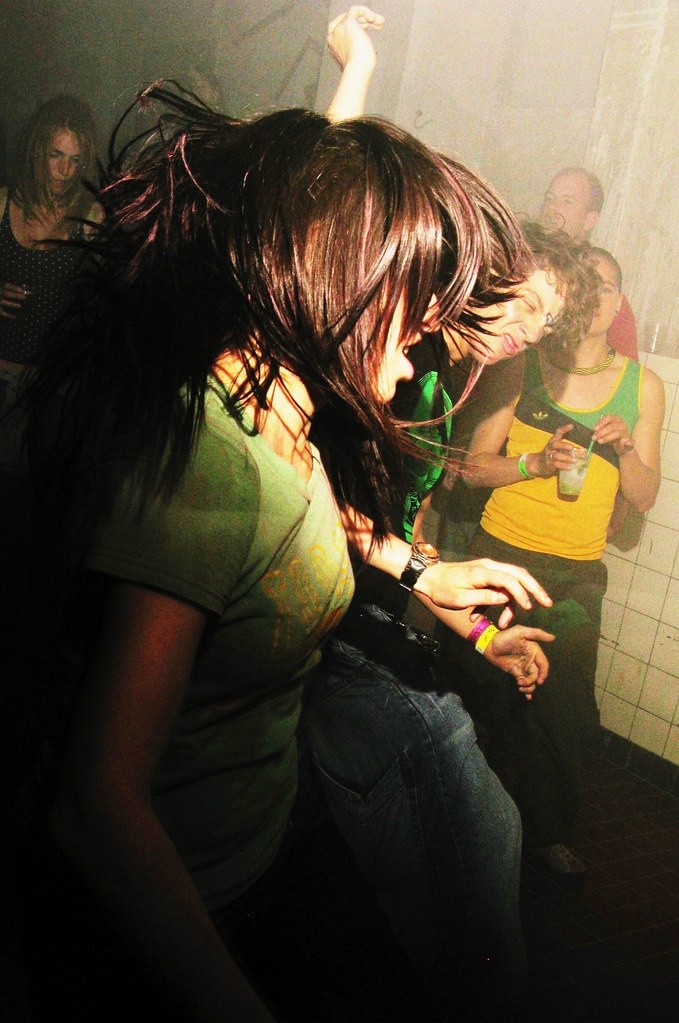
[399,541,438,591]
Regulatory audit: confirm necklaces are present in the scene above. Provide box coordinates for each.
[547,343,615,376]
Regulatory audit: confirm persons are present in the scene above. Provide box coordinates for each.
[0,6,664,1023]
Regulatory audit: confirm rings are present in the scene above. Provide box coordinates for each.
[550,451,555,461]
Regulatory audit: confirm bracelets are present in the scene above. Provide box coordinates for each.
[518,453,534,480]
[475,625,498,654]
[467,618,490,643]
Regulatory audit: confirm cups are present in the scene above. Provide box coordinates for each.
[3,281,36,318]
[558,447,590,496]
[646,321,668,354]
